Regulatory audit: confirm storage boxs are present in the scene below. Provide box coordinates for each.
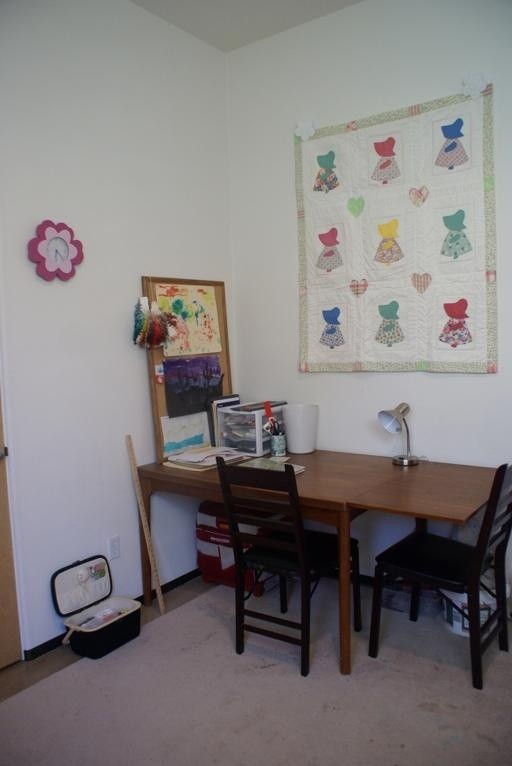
[217,400,284,458]
[49,553,143,661]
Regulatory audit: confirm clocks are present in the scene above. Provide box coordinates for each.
[25,218,85,282]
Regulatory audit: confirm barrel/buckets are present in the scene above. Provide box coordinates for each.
[283,404,319,455]
[440,586,511,638]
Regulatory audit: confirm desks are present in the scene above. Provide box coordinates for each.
[134,451,500,676]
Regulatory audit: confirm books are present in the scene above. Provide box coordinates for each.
[238,457,306,475]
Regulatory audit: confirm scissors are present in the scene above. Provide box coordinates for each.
[264,417,279,435]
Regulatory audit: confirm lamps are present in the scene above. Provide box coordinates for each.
[375,401,419,468]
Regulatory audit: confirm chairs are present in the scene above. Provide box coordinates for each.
[215,455,364,677]
[367,462,512,691]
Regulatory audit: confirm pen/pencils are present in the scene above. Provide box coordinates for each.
[279,432,283,435]
